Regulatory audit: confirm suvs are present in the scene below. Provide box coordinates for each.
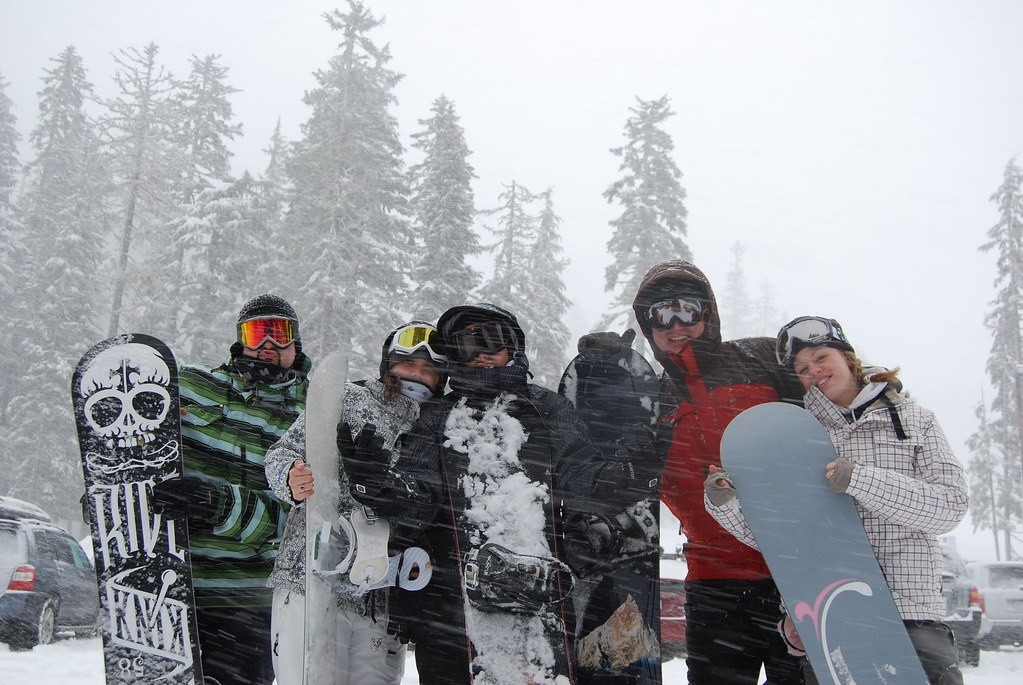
[0,494,102,652]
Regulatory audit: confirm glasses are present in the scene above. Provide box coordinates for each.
[392,324,450,364]
[241,313,298,351]
[644,298,707,330]
[450,321,510,362]
[776,317,833,366]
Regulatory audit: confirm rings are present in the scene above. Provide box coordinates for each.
[299,484,305,491]
[830,469,835,475]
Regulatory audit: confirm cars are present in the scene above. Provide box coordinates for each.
[963,561,1023,651]
[659,576,688,661]
[938,544,986,669]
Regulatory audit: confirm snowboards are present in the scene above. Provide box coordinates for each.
[558,347,663,685]
[302,350,349,685]
[70,332,205,685]
[440,389,573,685]
[719,402,932,685]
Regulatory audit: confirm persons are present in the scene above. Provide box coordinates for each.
[703,315,968,685]
[337,303,666,685]
[80,294,312,685]
[264,319,451,685]
[577,259,903,685]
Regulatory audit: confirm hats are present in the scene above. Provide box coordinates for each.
[790,319,853,368]
[643,279,705,309]
[237,293,297,323]
[388,323,445,376]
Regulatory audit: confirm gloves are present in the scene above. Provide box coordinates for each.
[335,421,392,505]
[149,476,228,523]
[578,327,636,365]
[598,423,659,503]
